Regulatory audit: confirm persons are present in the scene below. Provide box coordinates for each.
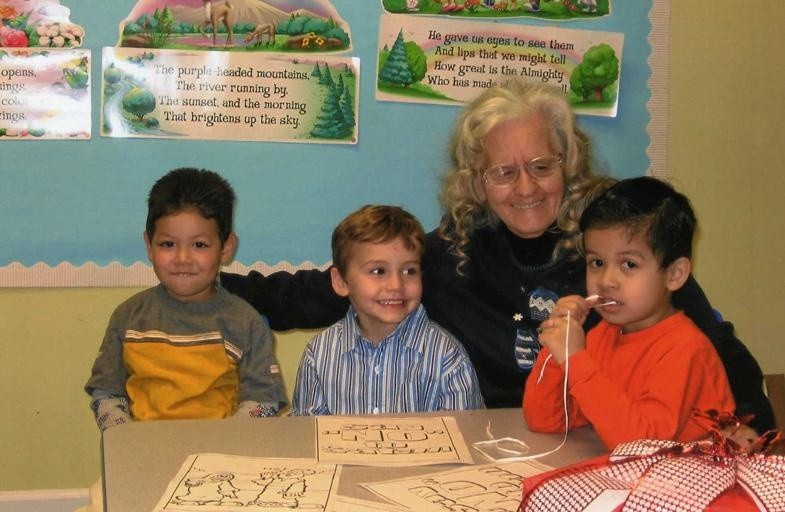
[216,76,781,457]
[69,166,292,512]
[520,175,738,457]
[282,203,491,415]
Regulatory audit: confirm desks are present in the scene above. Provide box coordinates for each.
[100,407,608,511]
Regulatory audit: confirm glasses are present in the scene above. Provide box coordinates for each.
[480,152,564,189]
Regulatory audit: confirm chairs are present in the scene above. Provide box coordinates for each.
[759,373,784,456]
[271,324,328,415]
